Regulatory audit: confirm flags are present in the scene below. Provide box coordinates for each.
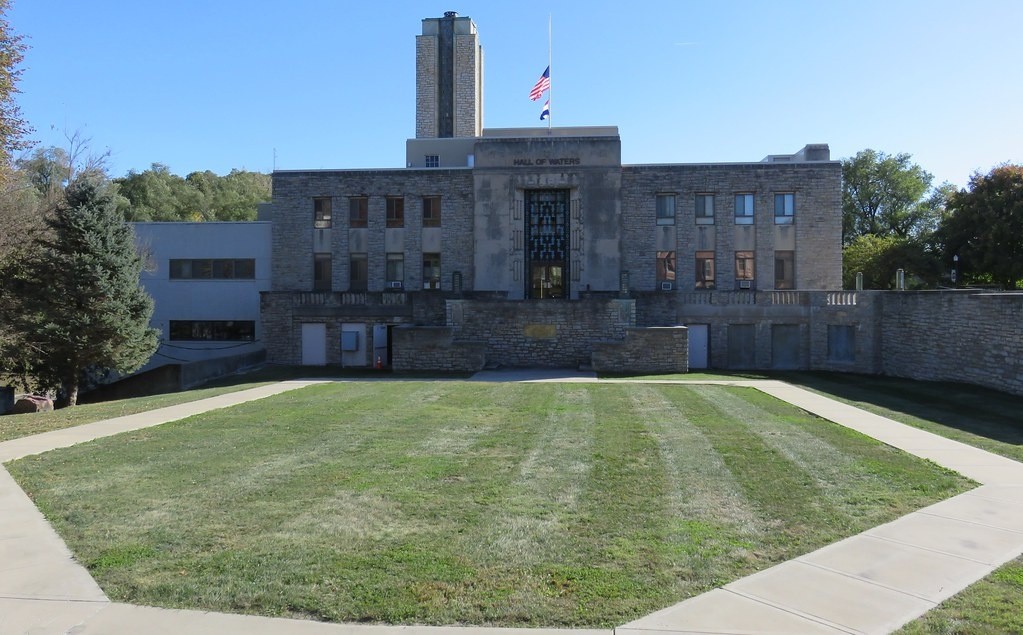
[539,100,549,120]
[529,66,550,101]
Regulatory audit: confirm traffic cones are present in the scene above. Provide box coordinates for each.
[376,355,383,368]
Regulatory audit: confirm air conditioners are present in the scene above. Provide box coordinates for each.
[392,282,401,288]
[661,282,671,290]
[740,281,750,288]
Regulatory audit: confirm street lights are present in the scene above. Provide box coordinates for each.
[953,254,959,283]
